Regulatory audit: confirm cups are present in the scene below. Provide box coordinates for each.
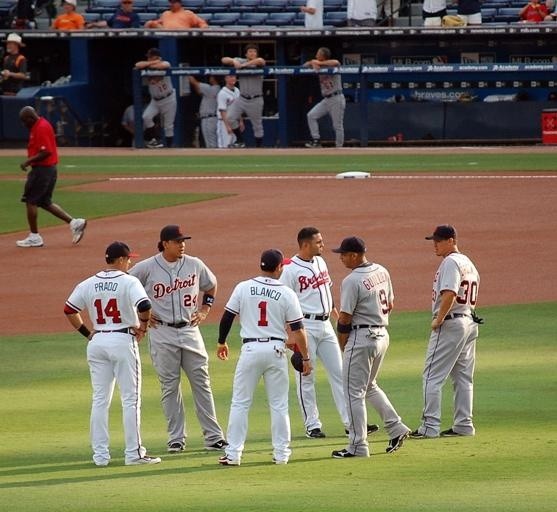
[388,132,403,143]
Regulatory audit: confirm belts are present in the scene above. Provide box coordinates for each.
[242,336,286,344]
[153,319,188,328]
[240,94,262,99]
[324,90,341,99]
[303,313,329,321]
[95,327,137,336]
[445,313,468,320]
[350,324,385,330]
[199,114,217,120]
[152,90,173,102]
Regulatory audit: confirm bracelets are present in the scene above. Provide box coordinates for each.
[77,325,91,337]
[140,315,150,325]
[201,292,213,306]
[301,356,311,361]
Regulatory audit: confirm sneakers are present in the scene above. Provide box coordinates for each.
[271,457,289,465]
[440,427,460,437]
[227,143,245,148]
[15,232,44,248]
[68,217,88,245]
[304,427,327,439]
[123,455,162,467]
[332,423,411,459]
[167,442,242,466]
[408,428,424,439]
[304,139,322,148]
[145,139,163,149]
[92,454,110,467]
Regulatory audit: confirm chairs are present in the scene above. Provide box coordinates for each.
[0,0,557,25]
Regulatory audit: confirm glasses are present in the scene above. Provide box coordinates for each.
[121,1,130,4]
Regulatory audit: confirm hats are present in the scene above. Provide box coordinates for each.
[144,47,162,58]
[160,223,192,242]
[260,247,291,269]
[61,0,78,11]
[331,237,366,254]
[104,240,139,258]
[424,224,457,241]
[1,32,26,48]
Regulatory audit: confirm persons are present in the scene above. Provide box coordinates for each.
[216,250,311,466]
[408,225,483,439]
[299,0,556,27]
[1,31,31,96]
[331,236,412,457]
[129,226,229,451]
[63,241,162,468]
[300,47,346,147]
[38,44,267,147]
[1,0,208,34]
[16,107,89,248]
[279,226,379,437]
